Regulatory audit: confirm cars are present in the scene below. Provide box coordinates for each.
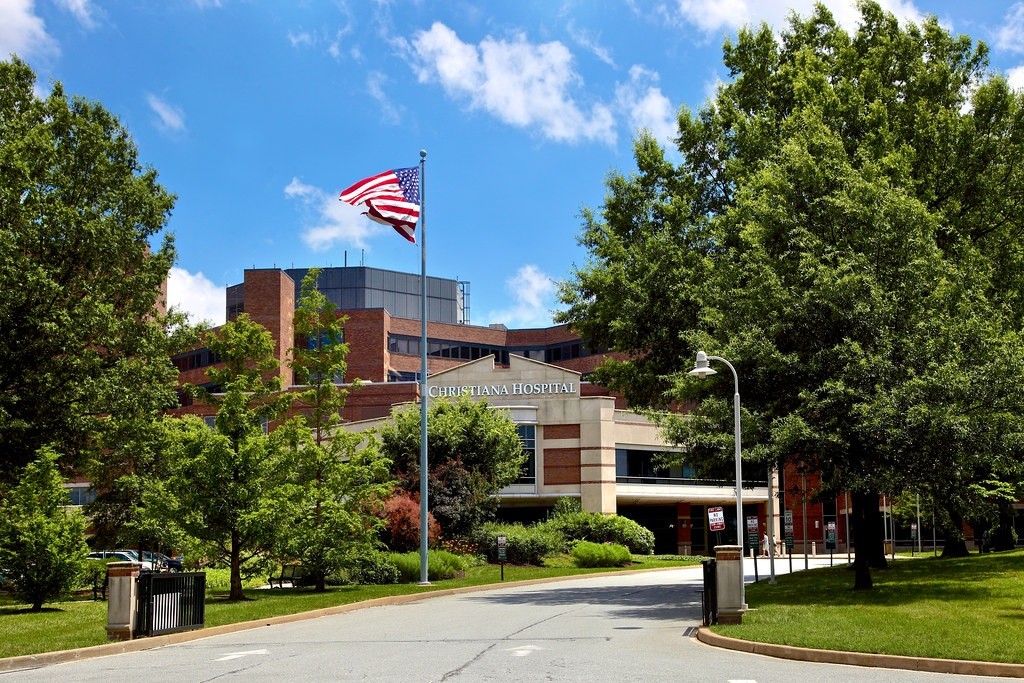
[87,547,184,573]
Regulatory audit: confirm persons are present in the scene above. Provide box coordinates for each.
[762,531,780,557]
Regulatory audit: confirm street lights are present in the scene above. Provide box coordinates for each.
[688,350,745,604]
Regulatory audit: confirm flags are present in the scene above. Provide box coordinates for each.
[338,165,420,246]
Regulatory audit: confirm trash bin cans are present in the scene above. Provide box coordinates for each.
[884,539,895,555]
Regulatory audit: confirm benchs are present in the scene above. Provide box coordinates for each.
[267,564,310,588]
[93,569,109,601]
